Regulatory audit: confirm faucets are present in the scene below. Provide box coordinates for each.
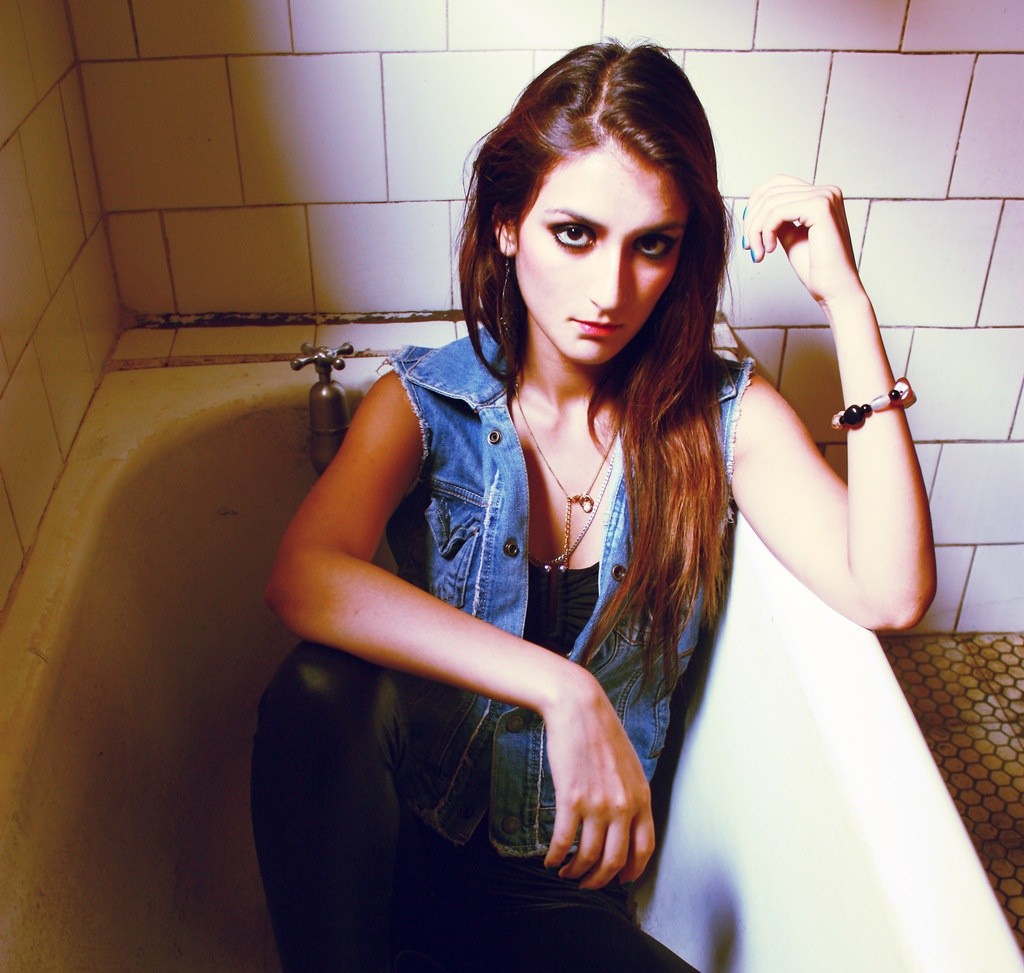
[289,341,355,478]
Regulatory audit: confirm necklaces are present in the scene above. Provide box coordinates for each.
[509,367,621,575]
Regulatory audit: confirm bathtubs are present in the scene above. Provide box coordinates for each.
[0,314,1024,973]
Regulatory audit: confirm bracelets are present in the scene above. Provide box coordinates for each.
[829,374,916,431]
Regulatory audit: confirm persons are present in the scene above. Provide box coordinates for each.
[245,44,941,970]
